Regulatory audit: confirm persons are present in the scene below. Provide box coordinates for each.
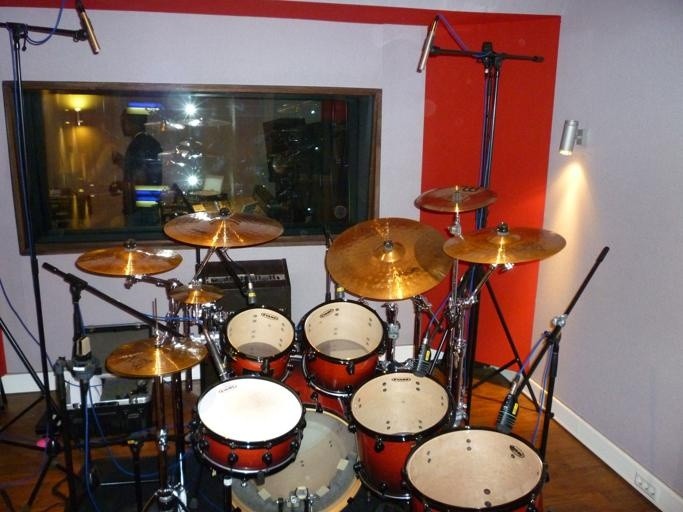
[110,108,163,228]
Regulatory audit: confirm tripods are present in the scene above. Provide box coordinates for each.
[0,22,154,512]
[430,46,540,429]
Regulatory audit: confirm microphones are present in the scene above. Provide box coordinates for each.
[75,1,100,54]
[248,282,259,308]
[411,329,432,375]
[201,329,230,382]
[416,18,438,73]
[497,394,519,433]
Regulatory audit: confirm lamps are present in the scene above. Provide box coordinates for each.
[559,118,585,158]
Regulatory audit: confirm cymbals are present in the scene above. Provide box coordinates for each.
[444,227,566,264]
[416,186,497,213]
[165,210,283,247]
[107,338,209,377]
[170,285,222,306]
[326,218,452,302]
[76,245,181,276]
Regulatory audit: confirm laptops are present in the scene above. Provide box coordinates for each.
[188,172,224,198]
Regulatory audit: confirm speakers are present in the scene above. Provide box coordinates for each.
[196,258,291,394]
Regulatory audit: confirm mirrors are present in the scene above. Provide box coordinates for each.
[2,79,382,256]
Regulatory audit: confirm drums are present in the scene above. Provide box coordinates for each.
[407,426,546,511]
[299,300,389,399]
[350,373,454,502]
[222,308,296,384]
[195,377,305,479]
[226,405,361,511]
